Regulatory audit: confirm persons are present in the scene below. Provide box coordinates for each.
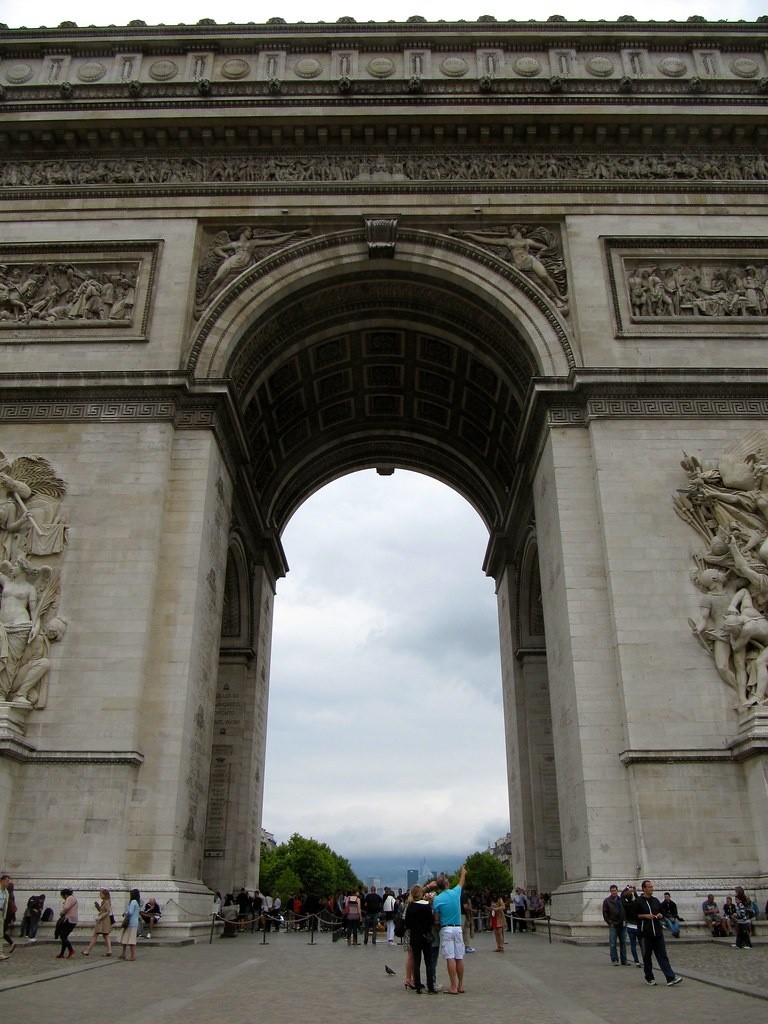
[209,885,406,944]
[198,226,296,308]
[0,265,137,325]
[18,894,45,942]
[693,464,768,711]
[0,473,68,699]
[0,875,17,960]
[602,880,682,986]
[628,262,768,316]
[461,224,569,307]
[401,865,481,995]
[81,889,112,955]
[56,889,78,959]
[703,887,758,948]
[0,153,768,186]
[139,898,161,938]
[478,884,551,952]
[117,889,140,961]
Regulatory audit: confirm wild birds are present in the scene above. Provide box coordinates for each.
[384,965,396,976]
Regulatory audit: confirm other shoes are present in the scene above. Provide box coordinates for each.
[126,958,135,961]
[623,962,632,966]
[147,933,151,939]
[67,950,75,958]
[744,946,751,949]
[613,961,619,966]
[30,938,38,941]
[81,951,89,956]
[635,963,643,967]
[465,947,476,952]
[0,954,9,960]
[118,955,126,959]
[731,944,740,949]
[103,953,112,956]
[404,981,465,995]
[9,944,17,953]
[56,954,64,958]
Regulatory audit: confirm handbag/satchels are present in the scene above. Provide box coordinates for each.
[423,933,435,944]
[109,907,115,924]
[120,915,129,929]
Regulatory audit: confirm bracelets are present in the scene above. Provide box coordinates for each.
[426,885,430,888]
[627,887,629,889]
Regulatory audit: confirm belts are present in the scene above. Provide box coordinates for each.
[442,924,460,927]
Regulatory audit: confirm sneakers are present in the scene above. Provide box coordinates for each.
[667,976,682,985]
[646,980,657,985]
